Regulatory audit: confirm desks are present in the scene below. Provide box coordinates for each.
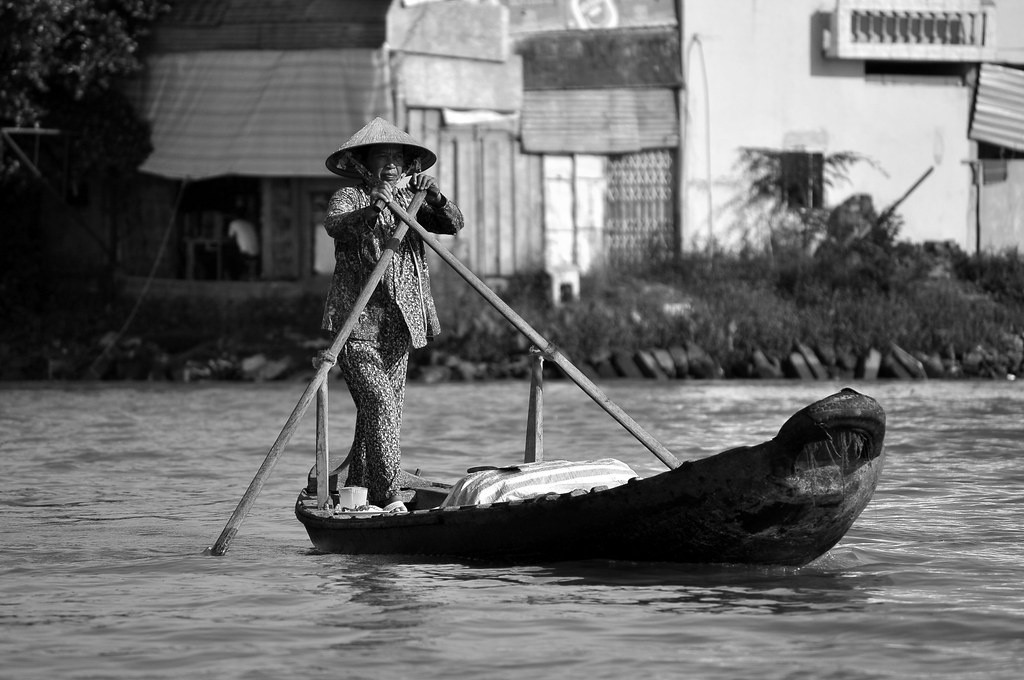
[185,234,231,282]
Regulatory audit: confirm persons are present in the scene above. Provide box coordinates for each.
[227,208,258,277]
[323,116,464,511]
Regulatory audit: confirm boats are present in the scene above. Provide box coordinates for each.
[294,388,887,568]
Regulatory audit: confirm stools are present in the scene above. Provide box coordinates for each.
[240,259,261,281]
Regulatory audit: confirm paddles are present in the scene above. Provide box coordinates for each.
[364,184,684,470]
[203,183,425,560]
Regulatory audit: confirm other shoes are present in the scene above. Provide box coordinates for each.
[383,501,408,515]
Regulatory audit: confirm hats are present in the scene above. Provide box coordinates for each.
[325,117,438,178]
